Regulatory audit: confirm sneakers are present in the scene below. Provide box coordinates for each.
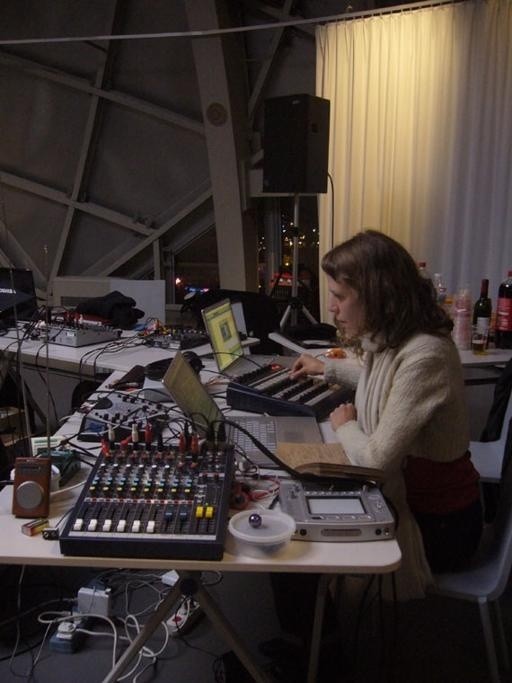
[257,633,358,683]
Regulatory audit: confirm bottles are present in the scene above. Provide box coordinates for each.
[418,262,492,355]
[494,270,512,348]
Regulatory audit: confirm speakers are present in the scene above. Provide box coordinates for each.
[261,94,332,194]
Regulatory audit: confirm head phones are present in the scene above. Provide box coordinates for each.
[43,304,74,324]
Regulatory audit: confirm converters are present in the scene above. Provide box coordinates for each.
[77,587,112,617]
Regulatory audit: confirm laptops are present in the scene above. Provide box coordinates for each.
[201,298,298,379]
[160,351,325,469]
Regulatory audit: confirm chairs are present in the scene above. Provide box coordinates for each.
[181,288,284,356]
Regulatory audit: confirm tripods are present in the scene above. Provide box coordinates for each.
[270,195,319,327]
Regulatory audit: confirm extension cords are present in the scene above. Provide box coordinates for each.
[50,611,88,653]
[167,600,200,637]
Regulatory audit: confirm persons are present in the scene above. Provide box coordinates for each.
[254,229,487,682]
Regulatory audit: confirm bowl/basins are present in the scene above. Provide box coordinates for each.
[228,509,296,557]
